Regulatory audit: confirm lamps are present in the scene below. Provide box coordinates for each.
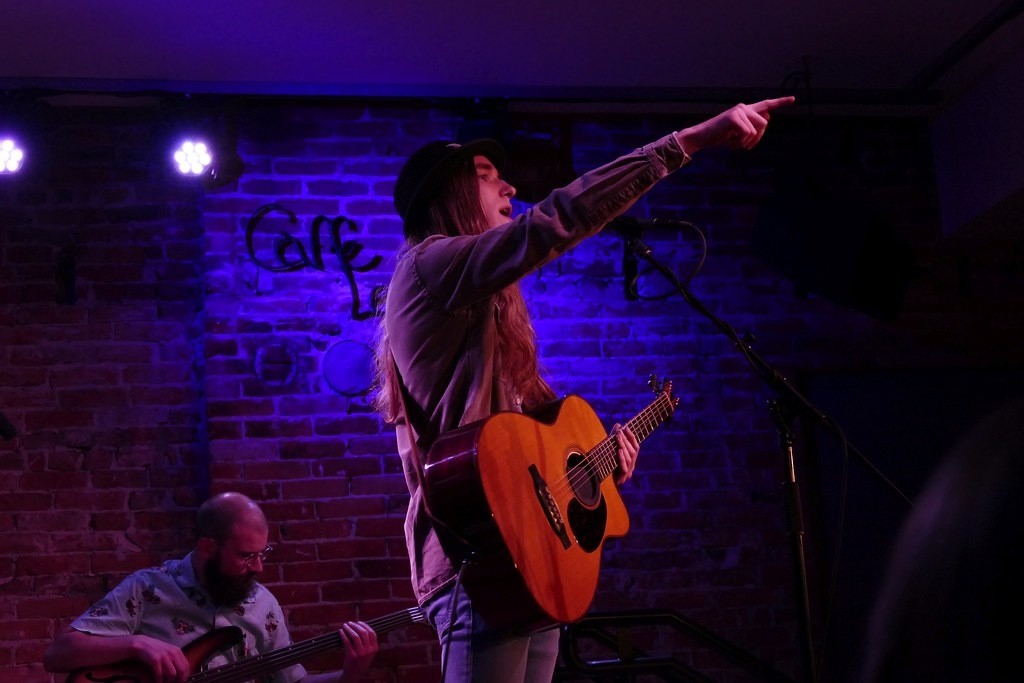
[1,139,25,175]
[171,135,216,180]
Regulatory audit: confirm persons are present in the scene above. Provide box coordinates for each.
[376,94,801,683]
[43,491,377,682]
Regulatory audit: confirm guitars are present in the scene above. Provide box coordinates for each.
[426,372,682,640]
[66,606,422,682]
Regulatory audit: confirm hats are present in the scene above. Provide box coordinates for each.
[394,139,487,212]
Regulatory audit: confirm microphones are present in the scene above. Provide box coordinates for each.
[600,217,689,239]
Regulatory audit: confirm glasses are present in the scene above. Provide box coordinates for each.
[212,537,273,566]
